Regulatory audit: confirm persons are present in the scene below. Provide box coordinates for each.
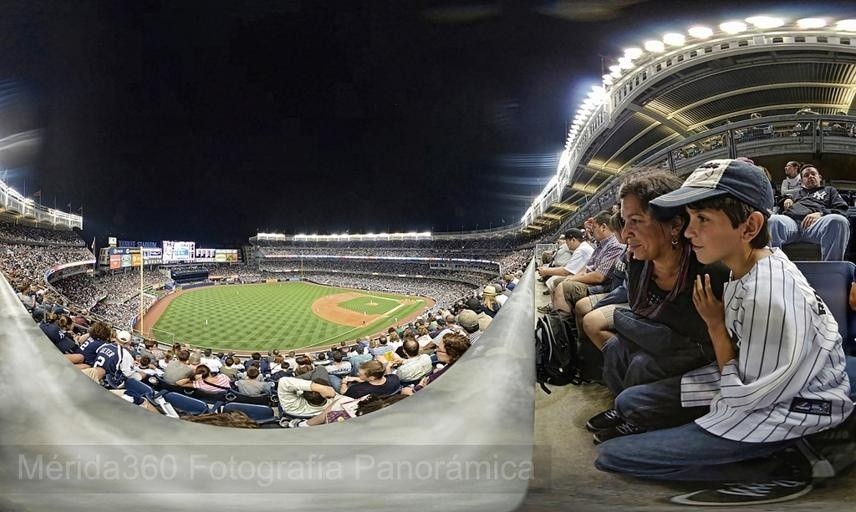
[0,105,856,505]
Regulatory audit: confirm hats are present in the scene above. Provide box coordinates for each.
[458,280,506,328]
[648,158,775,219]
[558,228,584,240]
[116,330,131,343]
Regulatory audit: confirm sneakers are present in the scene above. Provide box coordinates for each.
[537,304,553,313]
[592,423,647,443]
[585,410,619,431]
[670,477,812,506]
[788,437,836,479]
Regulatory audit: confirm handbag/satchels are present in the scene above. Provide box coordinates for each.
[533,308,588,395]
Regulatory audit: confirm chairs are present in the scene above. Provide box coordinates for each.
[66,341,360,431]
[773,230,856,433]
